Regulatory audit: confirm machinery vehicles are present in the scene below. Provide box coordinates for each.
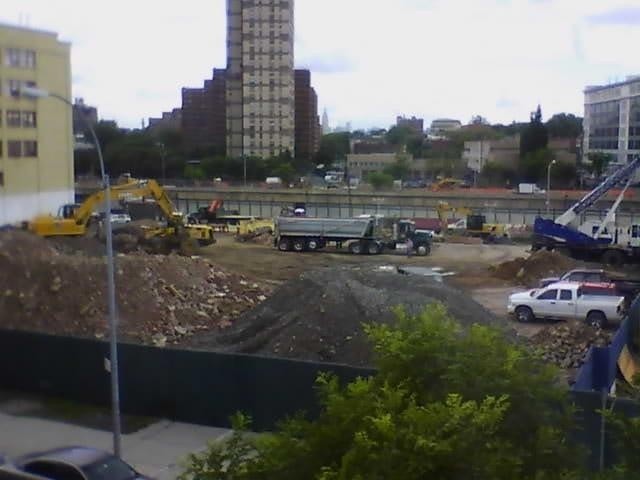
[531,153,640,261]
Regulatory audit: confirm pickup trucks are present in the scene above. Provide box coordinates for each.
[542,268,640,300]
[506,281,626,326]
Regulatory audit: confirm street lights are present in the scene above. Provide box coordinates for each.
[19,81,128,462]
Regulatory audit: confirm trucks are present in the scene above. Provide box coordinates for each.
[519,183,546,194]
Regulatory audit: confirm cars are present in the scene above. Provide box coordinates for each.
[1,442,156,480]
